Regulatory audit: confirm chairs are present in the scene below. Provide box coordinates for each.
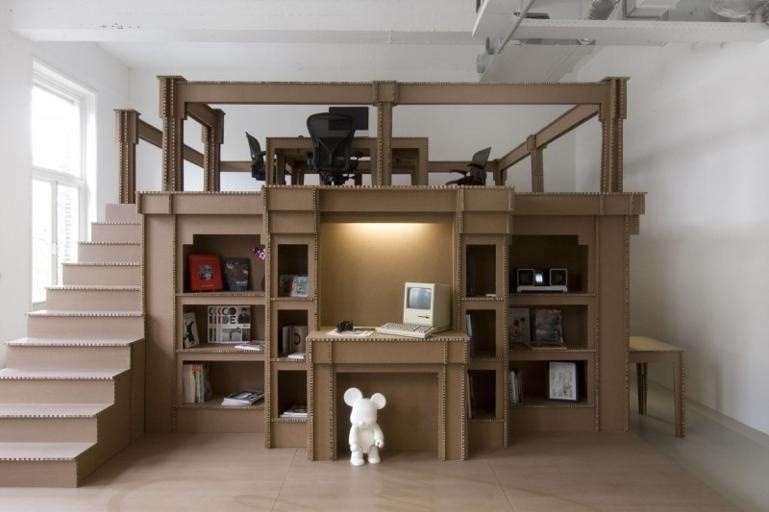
[302,112,363,187]
[444,146,492,186]
[243,131,292,184]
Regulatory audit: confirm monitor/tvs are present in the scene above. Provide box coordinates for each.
[403,282,452,333]
[329,107,368,131]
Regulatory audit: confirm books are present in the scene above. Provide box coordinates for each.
[182,306,265,352]
[467,373,473,420]
[183,363,264,408]
[278,273,309,298]
[466,313,474,361]
[510,307,569,351]
[280,404,308,418]
[282,324,308,359]
[509,366,525,406]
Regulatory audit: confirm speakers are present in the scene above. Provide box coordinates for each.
[550,268,568,285]
[517,268,535,287]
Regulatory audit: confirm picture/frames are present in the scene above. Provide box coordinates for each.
[509,307,569,351]
[546,361,578,403]
[189,254,222,292]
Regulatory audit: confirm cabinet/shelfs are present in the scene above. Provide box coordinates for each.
[508,234,597,436]
[459,235,508,447]
[172,235,264,431]
[264,234,316,449]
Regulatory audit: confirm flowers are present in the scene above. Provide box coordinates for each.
[248,243,267,262]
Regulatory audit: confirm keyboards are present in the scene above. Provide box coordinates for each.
[377,321,434,338]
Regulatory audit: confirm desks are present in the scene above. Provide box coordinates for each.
[308,326,470,462]
[626,334,687,438]
[264,137,430,187]
